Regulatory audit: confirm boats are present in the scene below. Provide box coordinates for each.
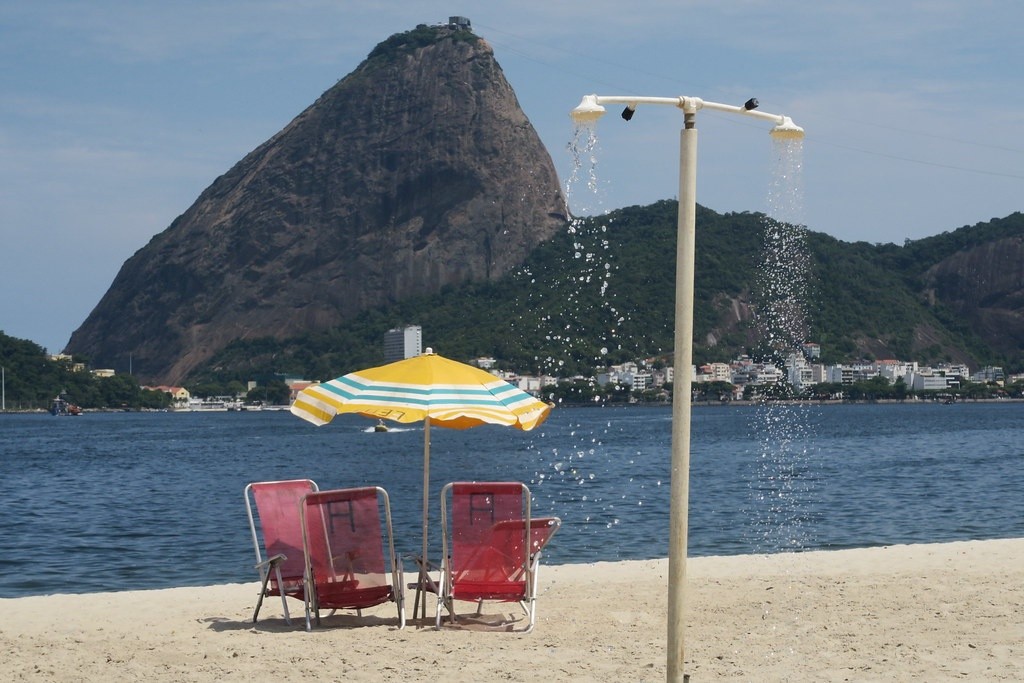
[375,425,388,432]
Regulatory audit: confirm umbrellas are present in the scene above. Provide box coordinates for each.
[290,346,554,624]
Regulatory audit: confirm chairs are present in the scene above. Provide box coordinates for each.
[405,515,564,621]
[298,486,406,633]
[244,479,362,628]
[434,481,539,633]
